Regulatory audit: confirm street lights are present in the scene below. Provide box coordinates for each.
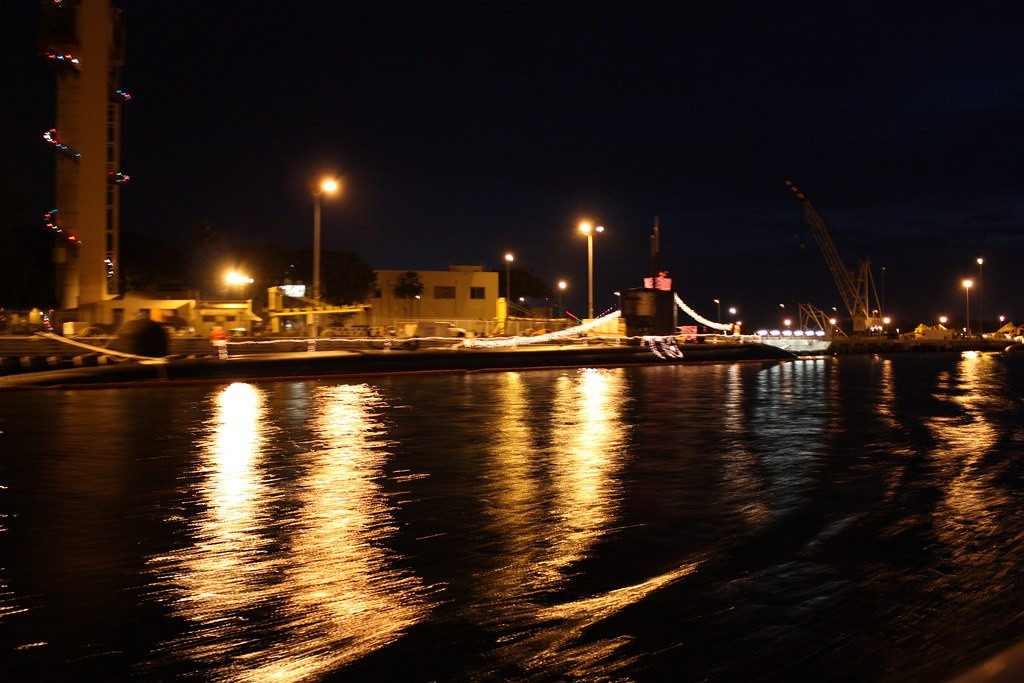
[976,258,983,334]
[881,267,886,317]
[713,299,721,334]
[557,280,568,319]
[308,178,339,338]
[579,223,594,319]
[963,279,973,336]
[505,254,514,319]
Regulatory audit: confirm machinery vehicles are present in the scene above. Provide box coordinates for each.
[784,180,892,337]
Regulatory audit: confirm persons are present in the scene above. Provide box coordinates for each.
[0,306,8,330]
[43,308,63,336]
